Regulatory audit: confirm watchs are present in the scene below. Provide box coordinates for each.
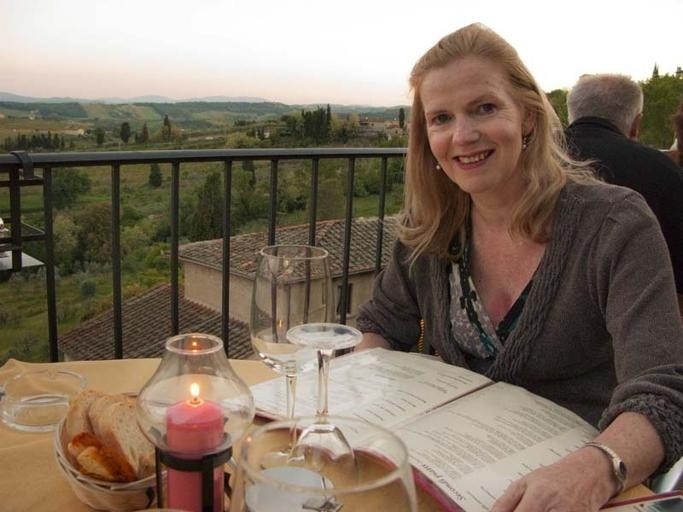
[586,440,629,494]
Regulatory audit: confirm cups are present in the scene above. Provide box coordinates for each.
[249,245,336,475]
[230,416,416,511]
[286,324,363,487]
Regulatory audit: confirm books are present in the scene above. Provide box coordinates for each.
[222,344,597,511]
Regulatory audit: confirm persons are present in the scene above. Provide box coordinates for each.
[355,23,683,511]
[557,73,683,298]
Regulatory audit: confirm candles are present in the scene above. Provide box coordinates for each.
[167,384,224,512]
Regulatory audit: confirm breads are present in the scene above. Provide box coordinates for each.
[67,430,99,457]
[73,452,126,490]
[66,389,109,437]
[98,402,156,482]
[89,393,129,437]
